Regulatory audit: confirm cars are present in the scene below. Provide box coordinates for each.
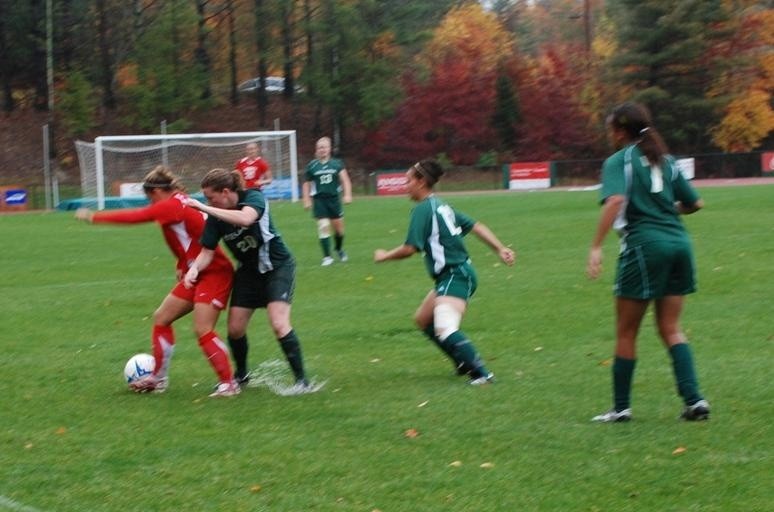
[237,74,304,94]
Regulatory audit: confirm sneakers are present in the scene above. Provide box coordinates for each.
[209,375,248,396]
[130,376,168,395]
[468,374,496,386]
[678,399,711,422]
[591,408,633,423]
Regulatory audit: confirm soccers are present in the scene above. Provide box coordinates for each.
[124,352,155,384]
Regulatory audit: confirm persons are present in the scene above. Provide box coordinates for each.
[184,166,315,397]
[302,136,353,267]
[585,102,711,422]
[372,158,515,386]
[74,165,242,397]
[233,143,273,192]
